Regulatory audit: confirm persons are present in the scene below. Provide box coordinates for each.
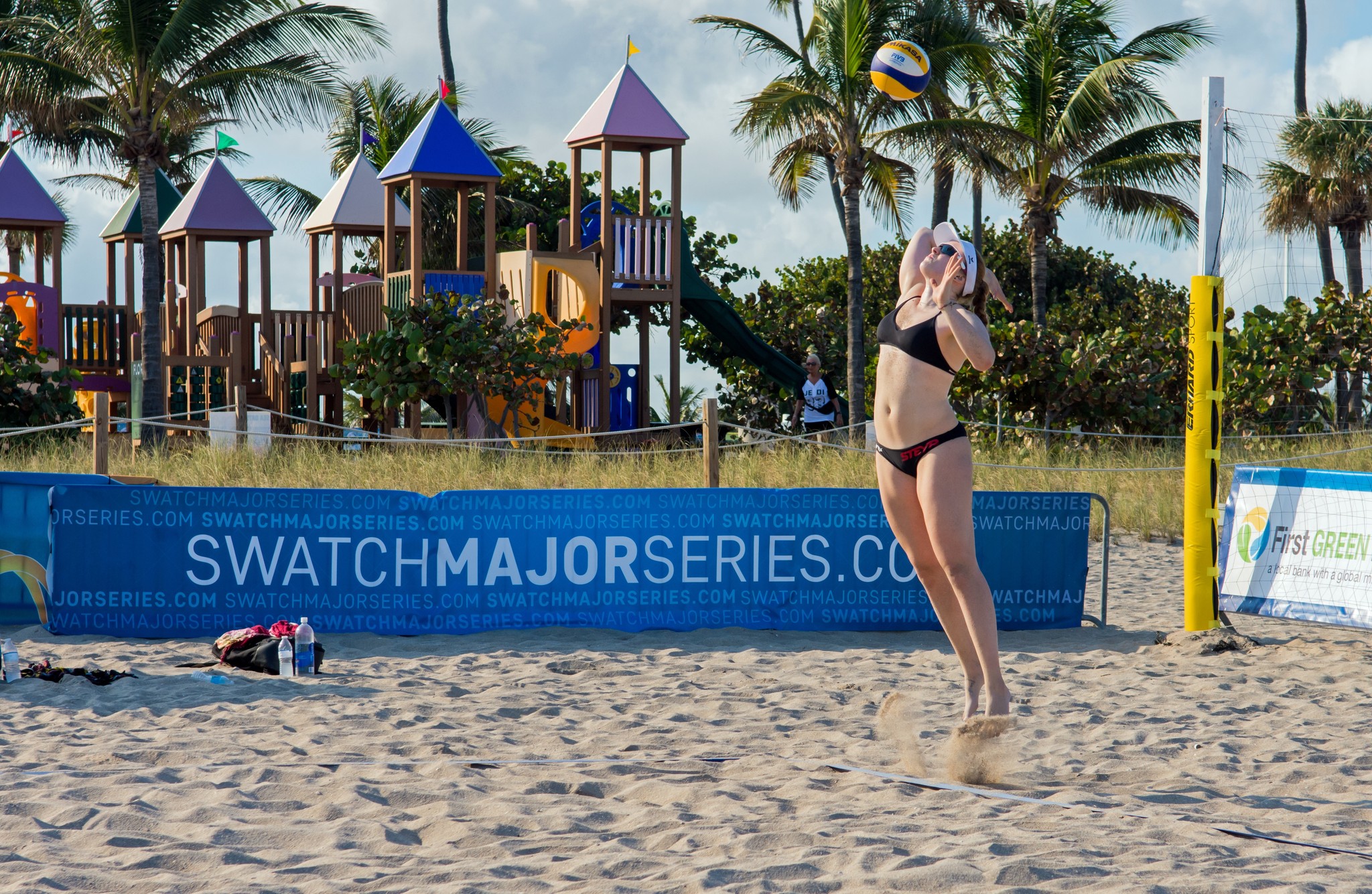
[868,222,1011,739]
[791,354,844,444]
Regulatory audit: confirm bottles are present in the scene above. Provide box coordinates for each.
[295,617,315,676]
[191,670,234,685]
[278,636,294,677]
[2,638,21,683]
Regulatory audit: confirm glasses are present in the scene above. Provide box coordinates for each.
[933,221,978,297]
[806,362,816,366]
[938,242,967,274]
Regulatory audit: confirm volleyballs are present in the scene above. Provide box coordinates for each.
[870,39,932,103]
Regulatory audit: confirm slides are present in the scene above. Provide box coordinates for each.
[482,345,598,450]
[676,222,874,434]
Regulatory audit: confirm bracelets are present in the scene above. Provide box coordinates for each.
[937,300,952,310]
[792,415,798,418]
[836,410,843,415]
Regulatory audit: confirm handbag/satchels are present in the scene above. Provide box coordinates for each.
[817,401,835,415]
[211,632,326,675]
